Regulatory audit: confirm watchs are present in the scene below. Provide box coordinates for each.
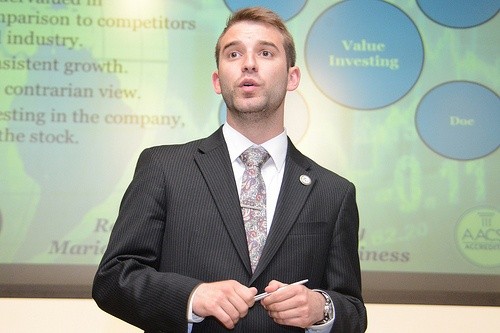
[313,290,333,325]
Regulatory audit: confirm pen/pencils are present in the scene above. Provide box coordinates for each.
[252,278,309,301]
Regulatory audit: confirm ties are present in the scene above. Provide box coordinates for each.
[239,148,271,274]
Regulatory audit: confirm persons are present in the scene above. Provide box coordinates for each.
[91,6,369,333]
